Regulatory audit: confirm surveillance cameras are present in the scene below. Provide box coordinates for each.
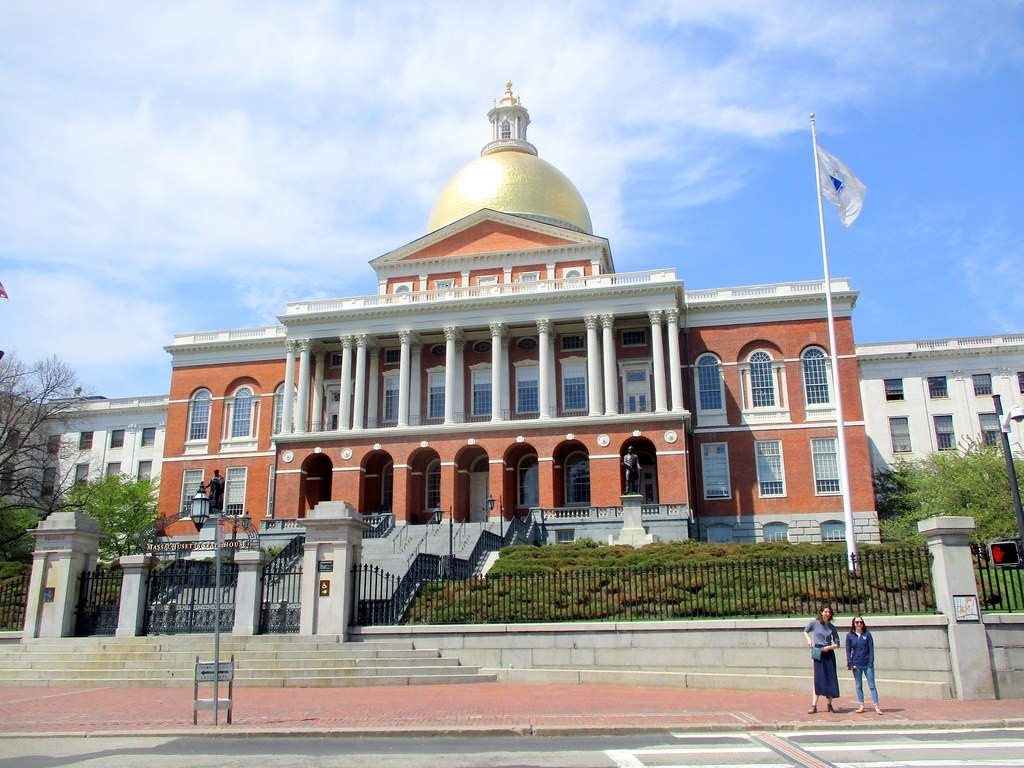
[1011,408,1024,422]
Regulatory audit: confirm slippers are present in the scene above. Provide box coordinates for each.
[857,708,865,713]
[875,708,883,715]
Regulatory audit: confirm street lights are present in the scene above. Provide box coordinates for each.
[486,494,504,549]
[191,480,221,727]
[991,394,1023,541]
[433,503,455,581]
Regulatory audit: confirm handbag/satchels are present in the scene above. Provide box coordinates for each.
[812,647,822,661]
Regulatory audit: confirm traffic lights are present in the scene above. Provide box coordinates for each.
[986,543,1020,570]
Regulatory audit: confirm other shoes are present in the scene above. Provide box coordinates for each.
[808,705,817,714]
[828,704,835,713]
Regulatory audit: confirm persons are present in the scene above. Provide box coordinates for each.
[804,605,840,714]
[205,469,225,513]
[624,446,643,494]
[846,616,883,714]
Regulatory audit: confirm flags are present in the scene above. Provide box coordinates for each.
[0,282,8,298]
[816,144,866,227]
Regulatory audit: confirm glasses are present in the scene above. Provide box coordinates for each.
[854,621,863,624]
